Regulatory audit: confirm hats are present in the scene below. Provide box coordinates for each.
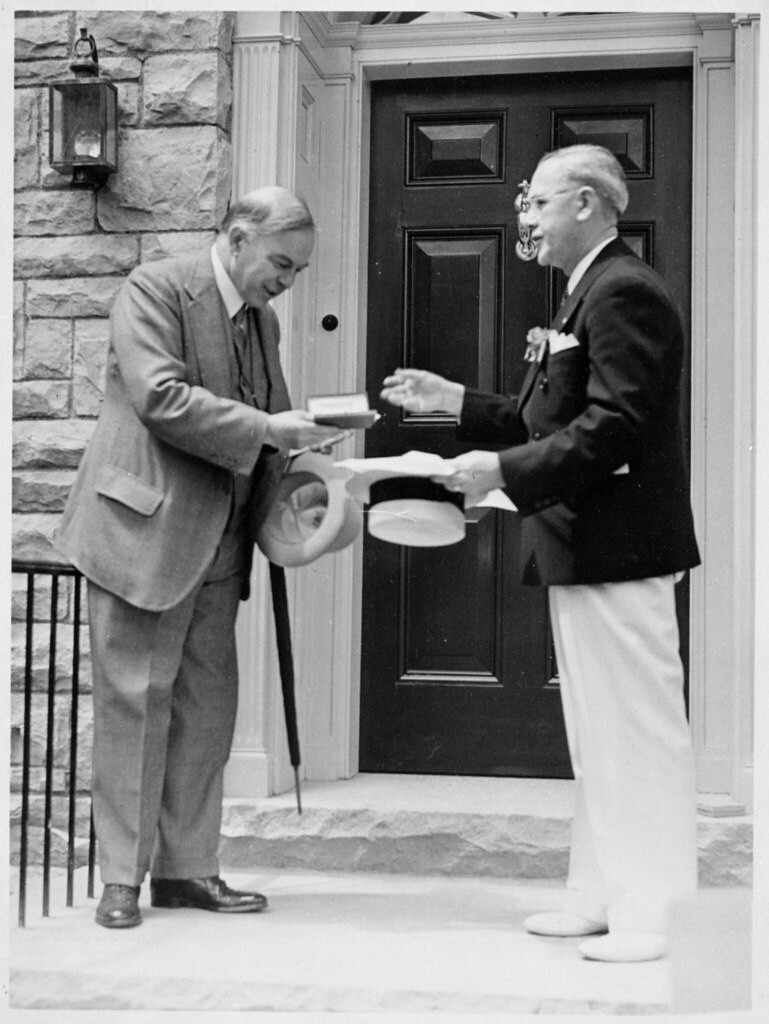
[254,450,365,568]
[330,451,517,547]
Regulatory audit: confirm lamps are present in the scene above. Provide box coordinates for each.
[45,27,118,189]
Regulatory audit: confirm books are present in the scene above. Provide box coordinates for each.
[305,390,378,428]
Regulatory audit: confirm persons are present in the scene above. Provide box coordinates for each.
[380,144,702,961]
[51,186,343,927]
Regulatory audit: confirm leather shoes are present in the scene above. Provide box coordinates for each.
[151,875,268,912]
[95,883,142,928]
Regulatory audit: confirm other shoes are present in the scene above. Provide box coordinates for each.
[580,930,668,963]
[525,907,607,937]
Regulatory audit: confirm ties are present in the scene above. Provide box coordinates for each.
[233,303,249,340]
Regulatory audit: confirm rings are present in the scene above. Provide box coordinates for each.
[454,486,461,491]
